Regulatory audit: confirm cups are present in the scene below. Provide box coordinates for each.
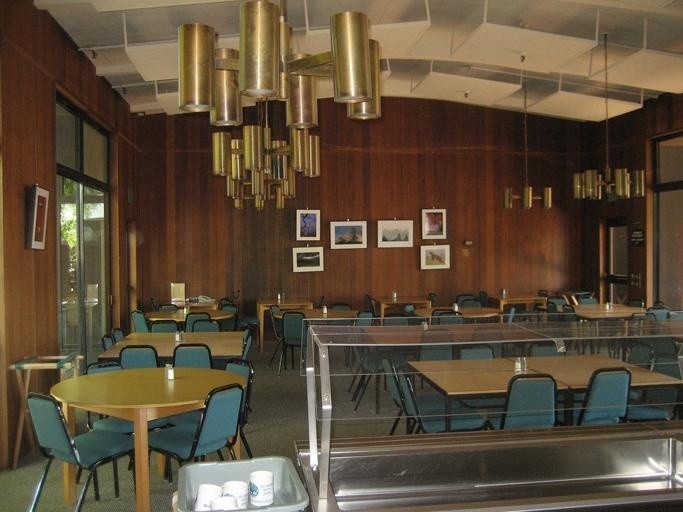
[195,471,275,512]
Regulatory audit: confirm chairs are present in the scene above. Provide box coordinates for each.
[261,289,681,436]
[30,295,262,512]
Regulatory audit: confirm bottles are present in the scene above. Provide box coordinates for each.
[193,297,199,304]
[501,287,508,297]
[392,288,396,299]
[453,302,458,314]
[277,292,281,299]
[183,305,189,313]
[606,302,611,310]
[175,330,182,342]
[421,319,427,329]
[321,303,327,314]
[164,362,174,380]
[512,353,527,372]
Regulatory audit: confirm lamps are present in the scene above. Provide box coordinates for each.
[503,55,555,211]
[570,32,644,203]
[165,0,387,213]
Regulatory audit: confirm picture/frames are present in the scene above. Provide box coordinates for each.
[421,207,447,240]
[27,184,49,250]
[291,247,324,272]
[329,220,367,249]
[418,243,450,271]
[376,219,415,248]
[295,209,321,241]
